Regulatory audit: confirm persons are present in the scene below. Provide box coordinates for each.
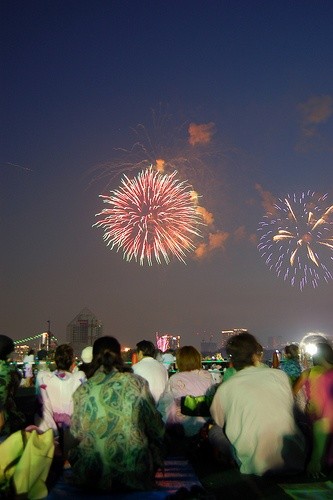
[0,333,333,500]
